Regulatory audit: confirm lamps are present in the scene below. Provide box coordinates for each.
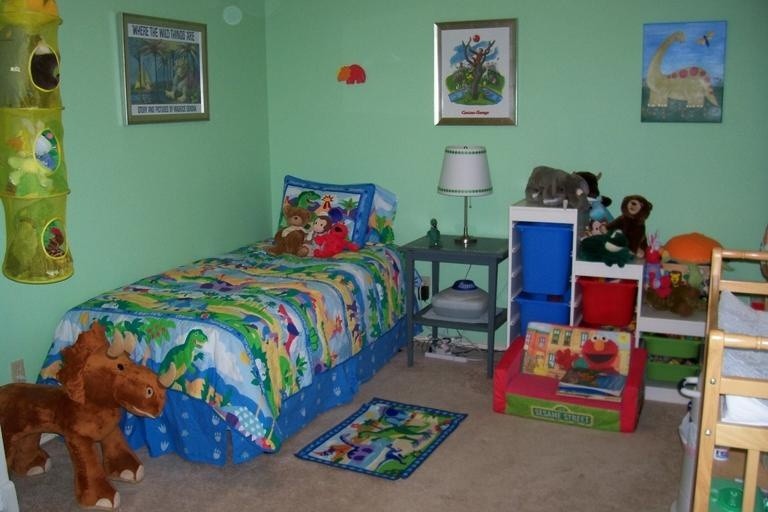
[437,145,493,245]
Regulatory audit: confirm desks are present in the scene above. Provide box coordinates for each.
[396,235,508,378]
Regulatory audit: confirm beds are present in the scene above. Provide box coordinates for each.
[689,237,768,512]
[36,176,424,467]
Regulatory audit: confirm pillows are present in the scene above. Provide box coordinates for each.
[368,183,397,231]
[278,175,375,250]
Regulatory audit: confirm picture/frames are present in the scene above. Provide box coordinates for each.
[641,20,727,122]
[115,11,209,126]
[433,19,517,126]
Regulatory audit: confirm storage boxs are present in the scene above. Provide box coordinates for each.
[514,282,571,337]
[646,361,702,383]
[514,222,572,295]
[576,276,638,326]
[640,336,702,360]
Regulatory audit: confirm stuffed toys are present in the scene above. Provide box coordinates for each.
[579,219,608,242]
[8,118,52,197]
[6,199,68,278]
[525,165,592,213]
[644,270,710,316]
[607,194,653,258]
[579,227,633,268]
[659,232,735,285]
[589,201,615,224]
[268,205,311,257]
[644,230,664,288]
[574,170,611,207]
[313,222,358,258]
[0,23,62,108]
[0,317,176,509]
[302,216,332,257]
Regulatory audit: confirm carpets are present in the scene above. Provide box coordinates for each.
[292,396,468,480]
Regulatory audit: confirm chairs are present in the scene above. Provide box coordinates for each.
[494,321,646,435]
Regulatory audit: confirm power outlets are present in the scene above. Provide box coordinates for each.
[420,277,432,291]
[12,360,28,383]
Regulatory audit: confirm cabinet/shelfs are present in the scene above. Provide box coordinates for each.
[507,198,711,405]
